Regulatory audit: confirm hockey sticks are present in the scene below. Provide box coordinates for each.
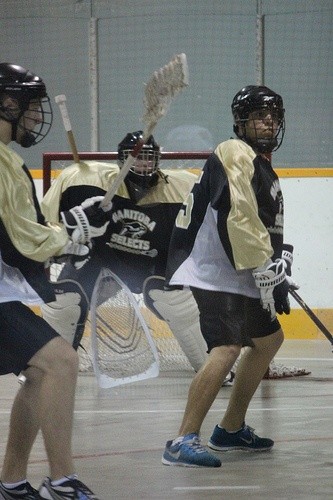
[287,286,333,344]
[101,52,190,210]
[55,95,160,389]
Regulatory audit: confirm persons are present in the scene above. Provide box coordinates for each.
[0,63,113,500]
[161,86,300,468]
[17,130,237,385]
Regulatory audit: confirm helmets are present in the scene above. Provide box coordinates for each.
[118,130,159,189]
[231,85,285,152]
[0,63,53,148]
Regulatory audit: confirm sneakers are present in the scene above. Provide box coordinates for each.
[161,433,222,467]
[38,474,99,500]
[207,422,274,452]
[0,480,46,500]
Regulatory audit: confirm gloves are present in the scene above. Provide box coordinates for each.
[45,238,96,269]
[252,258,300,315]
[60,196,113,246]
[281,244,294,277]
